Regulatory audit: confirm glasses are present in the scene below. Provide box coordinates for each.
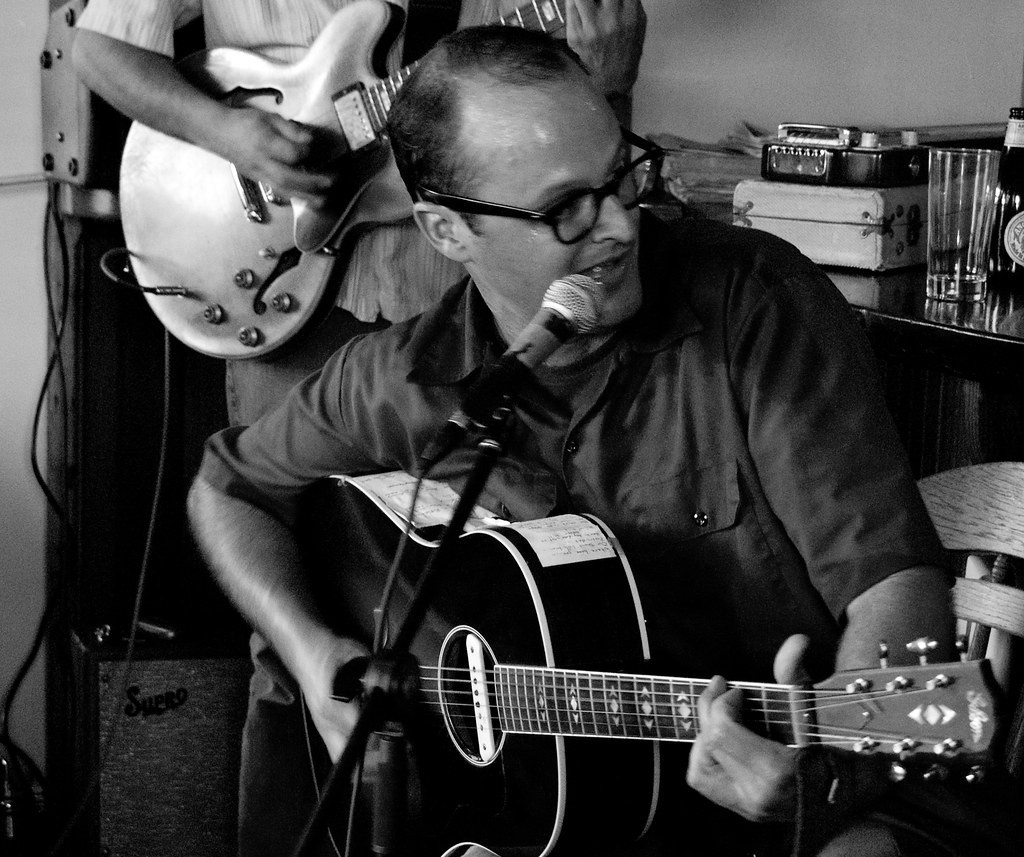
[412,130,665,245]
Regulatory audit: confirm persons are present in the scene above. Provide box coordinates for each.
[187,24,957,857]
[68,0,649,857]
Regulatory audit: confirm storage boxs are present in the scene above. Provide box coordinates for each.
[733,174,994,272]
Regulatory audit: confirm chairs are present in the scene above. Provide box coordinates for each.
[917,462,1024,775]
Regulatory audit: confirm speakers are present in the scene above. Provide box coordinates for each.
[65,613,327,857]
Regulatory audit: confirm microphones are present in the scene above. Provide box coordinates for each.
[417,273,607,469]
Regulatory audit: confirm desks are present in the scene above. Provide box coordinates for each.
[820,247,1024,477]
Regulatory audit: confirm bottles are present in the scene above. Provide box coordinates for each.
[988,108,1024,311]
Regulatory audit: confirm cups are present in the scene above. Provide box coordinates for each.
[925,147,1001,302]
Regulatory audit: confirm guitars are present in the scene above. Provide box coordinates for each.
[292,466,1013,856]
[116,1,567,364]
